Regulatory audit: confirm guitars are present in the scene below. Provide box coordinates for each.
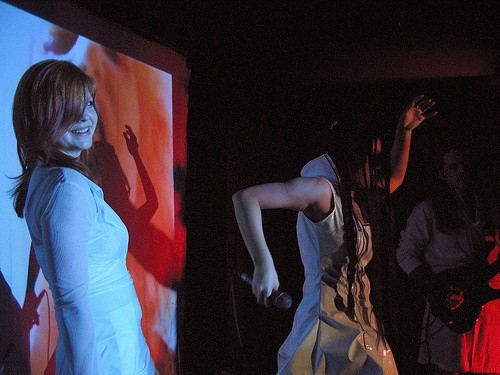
[436,242,500,333]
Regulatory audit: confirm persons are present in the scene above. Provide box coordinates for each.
[232,94,437,375]
[4,58,159,375]
[397,141,491,375]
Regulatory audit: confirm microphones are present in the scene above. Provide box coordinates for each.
[236,271,292,309]
